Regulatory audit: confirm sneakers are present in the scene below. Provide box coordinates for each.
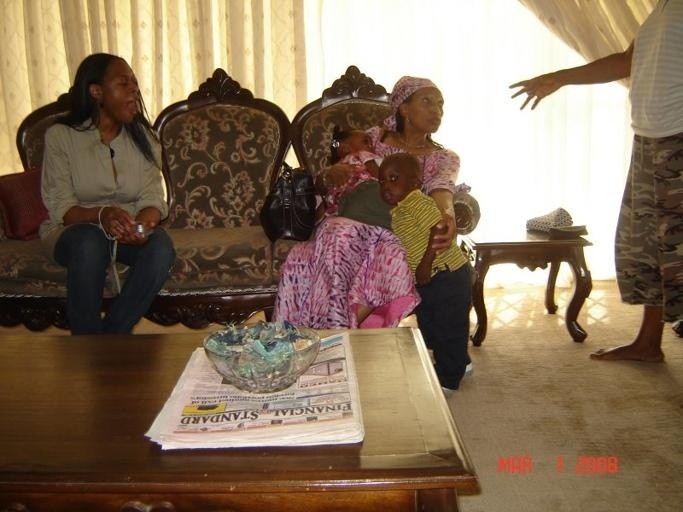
[464,363,472,378]
[440,386,456,400]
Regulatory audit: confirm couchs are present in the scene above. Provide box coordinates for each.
[0,64,482,332]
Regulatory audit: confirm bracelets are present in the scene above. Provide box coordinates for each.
[321,171,328,189]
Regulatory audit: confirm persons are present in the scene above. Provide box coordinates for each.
[36,53,176,336]
[269,75,460,329]
[324,123,397,228]
[506,0,682,364]
[377,152,479,399]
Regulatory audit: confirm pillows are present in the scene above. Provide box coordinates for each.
[0,166,49,241]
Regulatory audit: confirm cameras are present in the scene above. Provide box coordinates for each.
[119,223,146,244]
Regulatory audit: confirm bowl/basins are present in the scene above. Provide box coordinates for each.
[203,324,321,394]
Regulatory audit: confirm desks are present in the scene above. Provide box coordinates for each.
[461,228,595,348]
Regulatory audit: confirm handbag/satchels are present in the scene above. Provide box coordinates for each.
[261,166,315,241]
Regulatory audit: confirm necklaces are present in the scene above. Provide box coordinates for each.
[396,131,430,148]
